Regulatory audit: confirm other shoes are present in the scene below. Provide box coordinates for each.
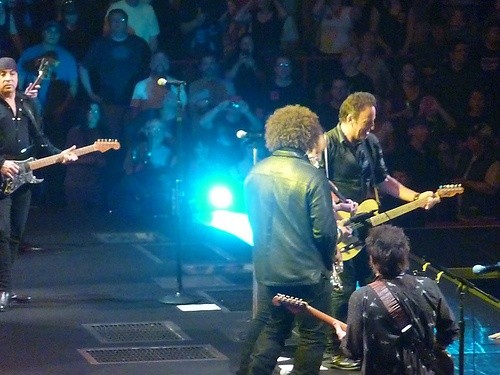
[330,355,361,370]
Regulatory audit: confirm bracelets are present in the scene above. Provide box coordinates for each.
[414,194,419,201]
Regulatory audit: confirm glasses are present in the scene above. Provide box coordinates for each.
[278,62,289,67]
[88,110,100,115]
[110,18,126,23]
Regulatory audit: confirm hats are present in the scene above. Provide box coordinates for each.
[0,57,17,72]
[339,47,360,65]
[473,123,492,137]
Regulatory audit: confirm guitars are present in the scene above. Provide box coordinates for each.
[0,138,121,200]
[271,292,348,332]
[334,184,464,261]
[27,56,61,94]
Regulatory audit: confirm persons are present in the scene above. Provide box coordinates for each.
[334,226,459,375]
[0,57,78,313]
[318,93,441,369]
[0,32,44,252]
[237,105,358,375]
[0,0,499,227]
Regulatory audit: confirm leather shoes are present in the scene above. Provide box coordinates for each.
[0,292,32,311]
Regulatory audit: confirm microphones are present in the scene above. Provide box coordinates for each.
[157,78,186,85]
[472,261,500,275]
[236,130,264,138]
